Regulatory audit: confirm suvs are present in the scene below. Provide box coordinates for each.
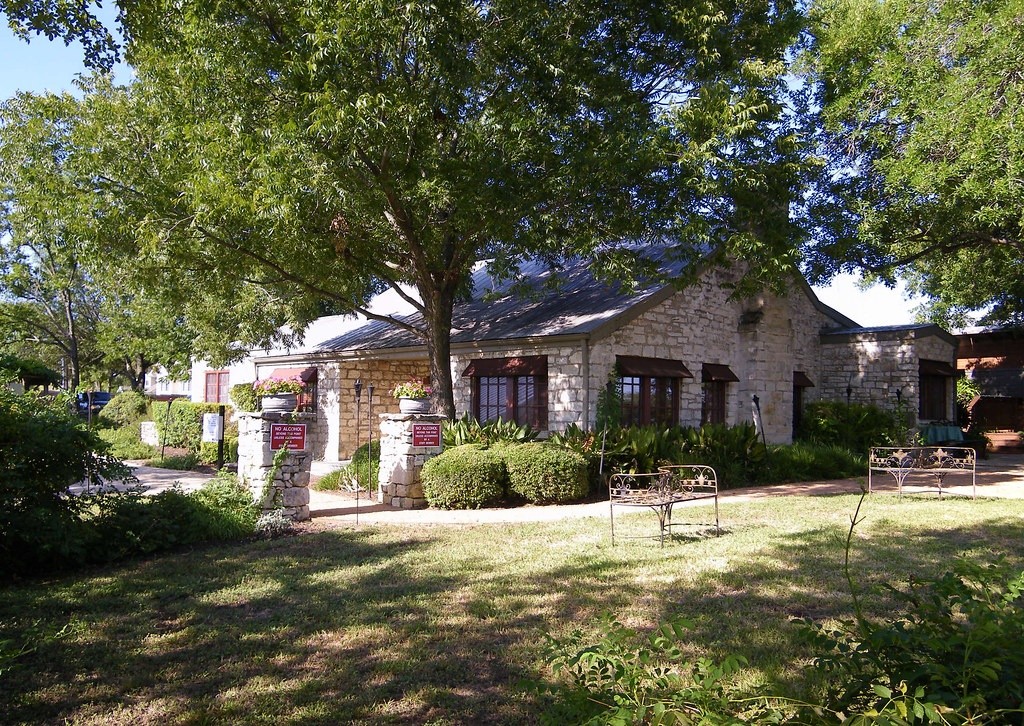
[78,392,113,420]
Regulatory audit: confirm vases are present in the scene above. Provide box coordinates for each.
[399,396,431,414]
[261,392,297,412]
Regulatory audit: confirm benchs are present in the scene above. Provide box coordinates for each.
[609,465,720,547]
[869,445,976,506]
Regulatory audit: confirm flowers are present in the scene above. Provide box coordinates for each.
[253,375,306,397]
[389,380,433,400]
